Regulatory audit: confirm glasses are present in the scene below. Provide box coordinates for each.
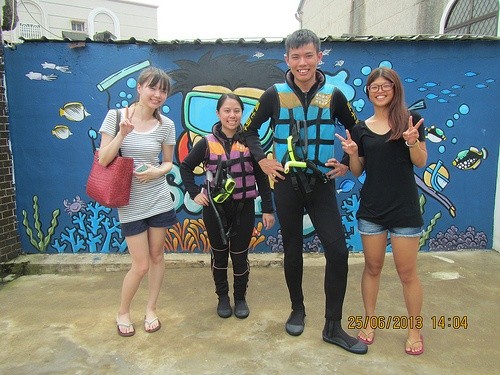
[368,83,395,93]
[211,177,236,203]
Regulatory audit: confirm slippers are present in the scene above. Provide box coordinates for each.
[406,333,424,355]
[144,315,161,332]
[116,318,135,337]
[357,329,376,345]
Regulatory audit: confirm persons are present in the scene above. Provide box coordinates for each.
[180,93,276,320]
[97,66,178,337]
[244,28,368,355]
[335,66,428,355]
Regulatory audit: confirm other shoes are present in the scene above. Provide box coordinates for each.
[286,308,305,336]
[217,296,232,318]
[234,298,250,319]
[323,320,368,354]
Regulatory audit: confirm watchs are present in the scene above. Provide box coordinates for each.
[405,139,420,148]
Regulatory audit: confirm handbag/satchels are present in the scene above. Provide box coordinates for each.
[85,109,134,208]
